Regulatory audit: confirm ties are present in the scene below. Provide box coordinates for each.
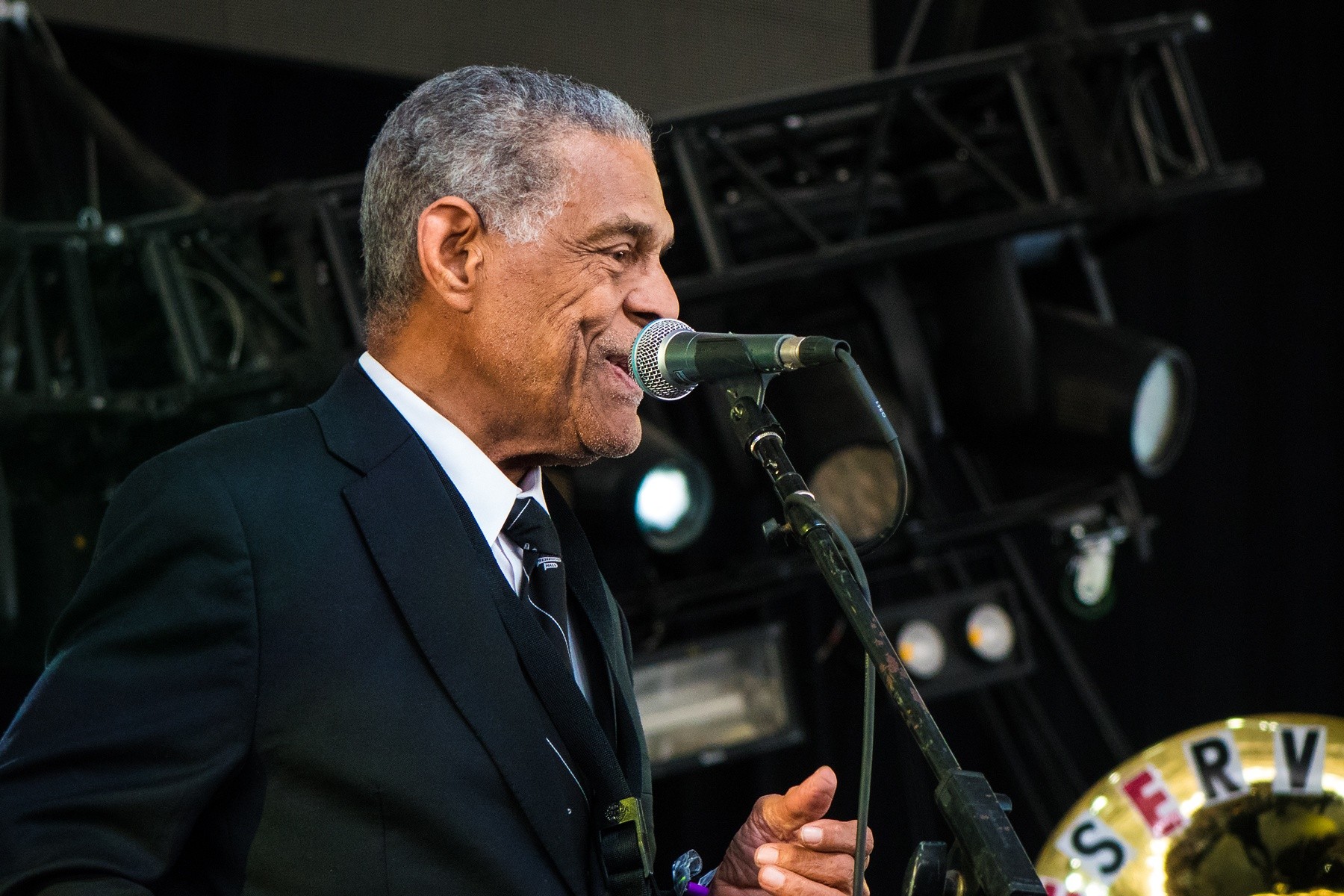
[501,497,574,673]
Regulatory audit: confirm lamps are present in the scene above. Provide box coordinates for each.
[569,299,1195,777]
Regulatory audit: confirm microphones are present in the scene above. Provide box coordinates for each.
[625,312,846,402]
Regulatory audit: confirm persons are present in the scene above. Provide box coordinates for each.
[0,65,876,896]
